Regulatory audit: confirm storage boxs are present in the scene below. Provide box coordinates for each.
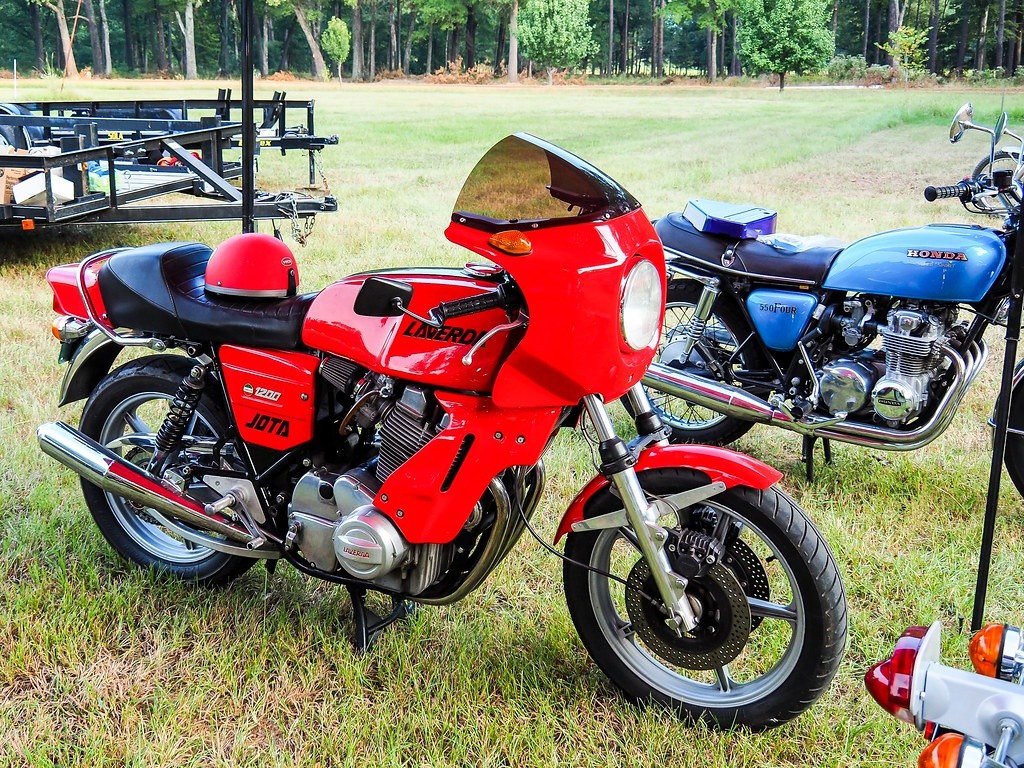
[0,144,74,207]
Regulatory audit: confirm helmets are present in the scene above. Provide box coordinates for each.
[206,232,300,297]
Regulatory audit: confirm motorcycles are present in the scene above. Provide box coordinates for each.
[38,130,850,739]
[612,104,1024,504]
